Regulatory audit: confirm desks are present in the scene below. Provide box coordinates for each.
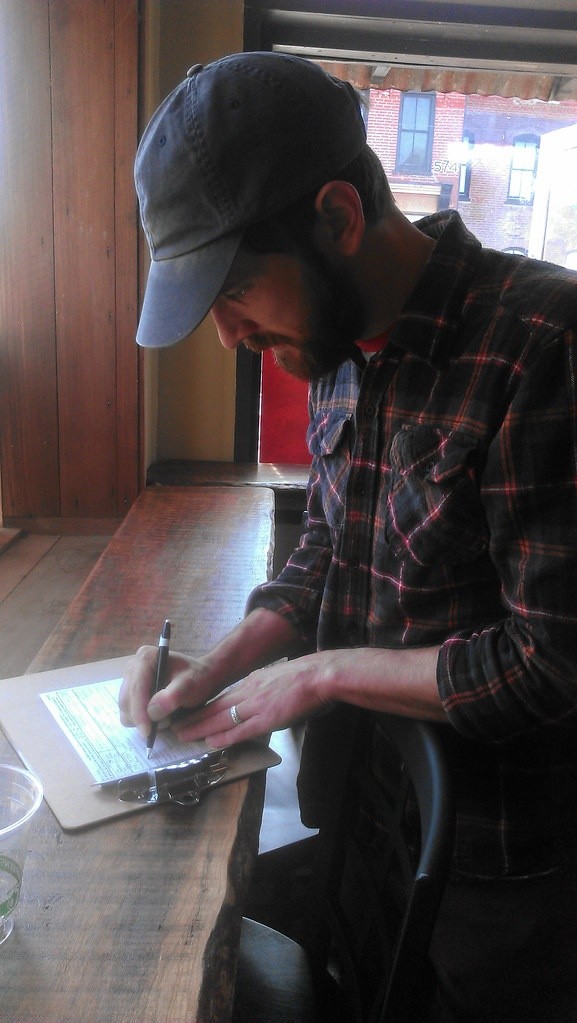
[1,484,281,1023]
[150,457,315,522]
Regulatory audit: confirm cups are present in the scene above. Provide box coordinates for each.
[0,764,44,942]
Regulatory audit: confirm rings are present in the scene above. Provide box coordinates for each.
[230,706,243,725]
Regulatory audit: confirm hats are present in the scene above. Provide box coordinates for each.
[132,50,369,350]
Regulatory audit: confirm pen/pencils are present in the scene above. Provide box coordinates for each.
[145,618,172,760]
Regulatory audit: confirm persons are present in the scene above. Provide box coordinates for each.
[114,51,577,1022]
